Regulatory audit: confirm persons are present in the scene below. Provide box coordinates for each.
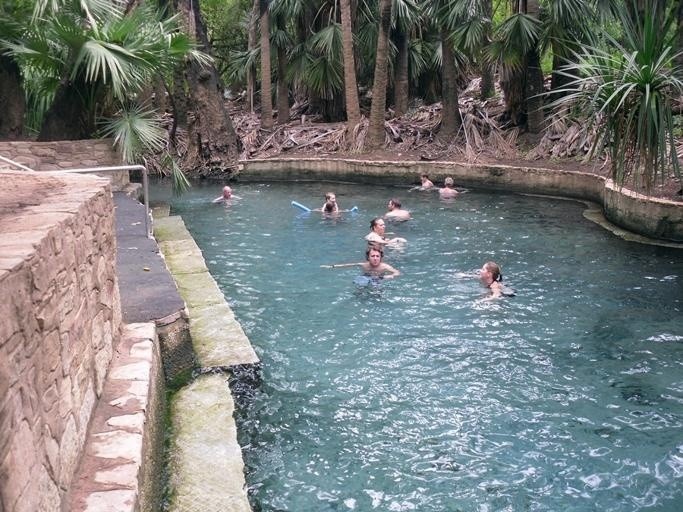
[213,186,239,208]
[460,262,503,307]
[384,198,410,222]
[365,218,407,253]
[315,192,338,220]
[409,172,459,198]
[320,245,400,279]
